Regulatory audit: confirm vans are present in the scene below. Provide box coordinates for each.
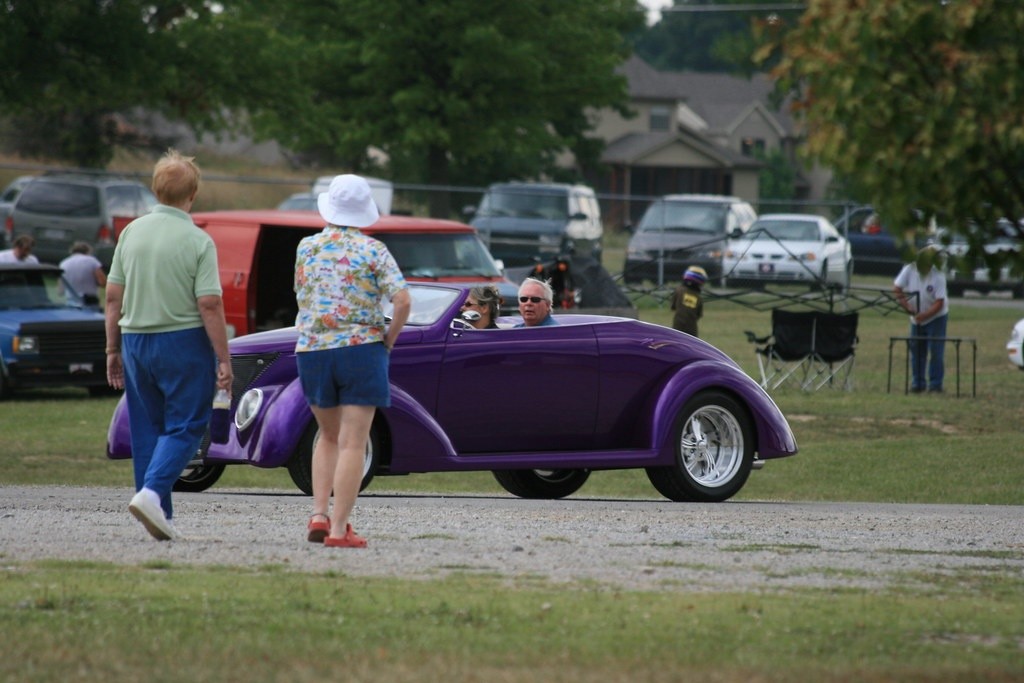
[621,191,757,296]
[462,183,604,278]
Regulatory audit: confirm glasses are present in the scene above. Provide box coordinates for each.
[519,297,547,303]
[461,301,478,307]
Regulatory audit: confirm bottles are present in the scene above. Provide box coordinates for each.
[210,389,231,444]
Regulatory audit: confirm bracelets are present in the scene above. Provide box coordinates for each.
[384,343,393,353]
[106,347,120,354]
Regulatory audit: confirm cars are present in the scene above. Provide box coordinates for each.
[186,208,523,345]
[834,205,943,272]
[926,215,1023,302]
[0,261,122,400]
[0,169,164,273]
[278,191,322,213]
[104,277,802,502]
[722,212,857,298]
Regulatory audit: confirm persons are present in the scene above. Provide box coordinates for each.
[57,241,107,307]
[460,286,501,329]
[671,266,708,337]
[105,150,234,542]
[0,235,39,265]
[514,277,561,327]
[293,174,411,549]
[894,261,949,392]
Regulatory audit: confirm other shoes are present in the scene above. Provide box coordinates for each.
[909,387,926,394]
[929,385,944,394]
[146,524,183,541]
[128,493,173,541]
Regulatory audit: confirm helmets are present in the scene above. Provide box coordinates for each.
[683,264,707,293]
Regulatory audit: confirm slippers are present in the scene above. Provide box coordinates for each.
[306,511,331,543]
[325,522,369,549]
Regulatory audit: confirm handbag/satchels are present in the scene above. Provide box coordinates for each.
[82,292,101,309]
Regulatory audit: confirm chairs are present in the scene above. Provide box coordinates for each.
[745,308,859,395]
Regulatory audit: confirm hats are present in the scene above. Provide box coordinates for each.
[317,173,379,227]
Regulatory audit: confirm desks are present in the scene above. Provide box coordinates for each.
[887,337,977,398]
